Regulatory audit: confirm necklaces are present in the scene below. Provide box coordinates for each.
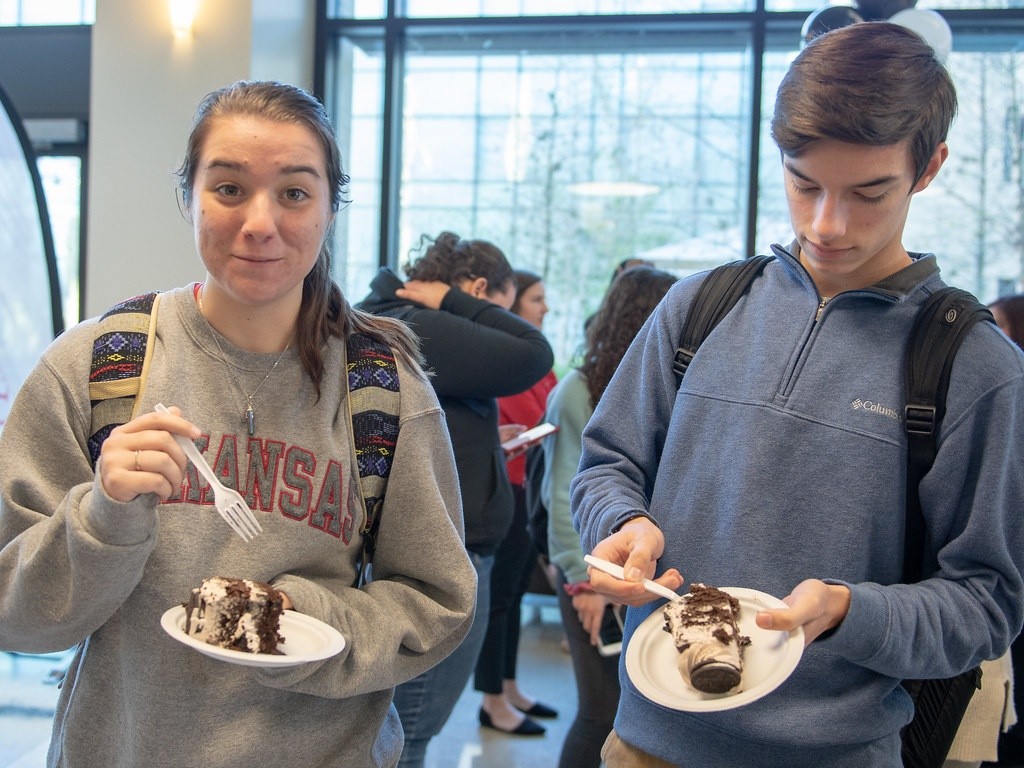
[199,285,296,435]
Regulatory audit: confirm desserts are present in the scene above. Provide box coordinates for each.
[662,583,752,699]
[181,574,283,656]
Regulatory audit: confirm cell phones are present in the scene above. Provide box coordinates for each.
[500,423,559,456]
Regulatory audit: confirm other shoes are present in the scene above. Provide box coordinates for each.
[514,702,558,717]
[479,706,546,734]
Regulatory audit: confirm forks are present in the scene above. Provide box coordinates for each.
[154,402,263,543]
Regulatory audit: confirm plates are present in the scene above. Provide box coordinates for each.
[160,604,347,669]
[625,586,804,712]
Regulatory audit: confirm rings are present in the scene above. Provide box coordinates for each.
[134,449,141,471]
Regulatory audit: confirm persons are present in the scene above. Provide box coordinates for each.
[570,21,1024,768]
[542,258,678,768]
[353,230,554,768]
[979,293,1024,768]
[0,83,477,768]
[473,270,560,734]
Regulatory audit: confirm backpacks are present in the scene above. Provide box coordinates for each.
[672,255,998,768]
